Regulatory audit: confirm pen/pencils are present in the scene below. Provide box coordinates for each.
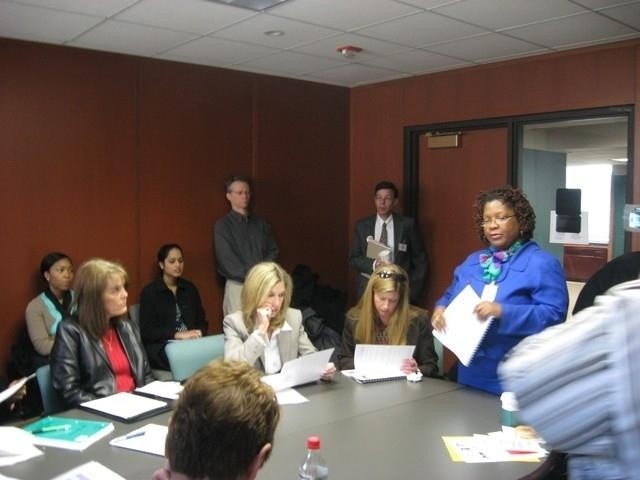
[127,432,145,438]
[31,425,64,433]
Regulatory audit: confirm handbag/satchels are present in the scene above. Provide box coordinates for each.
[302,307,341,370]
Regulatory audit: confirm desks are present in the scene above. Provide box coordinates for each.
[254,387,560,480]
[0,371,470,480]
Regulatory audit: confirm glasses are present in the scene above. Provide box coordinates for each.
[480,215,516,227]
[375,271,406,282]
[229,191,252,197]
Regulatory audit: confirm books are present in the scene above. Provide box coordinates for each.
[351,375,406,384]
[366,238,391,259]
[78,379,186,424]
[0,373,35,404]
[24,415,115,453]
[431,284,495,367]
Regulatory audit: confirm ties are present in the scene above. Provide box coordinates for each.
[379,222,387,245]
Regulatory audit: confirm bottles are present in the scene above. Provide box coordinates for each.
[297,436,329,480]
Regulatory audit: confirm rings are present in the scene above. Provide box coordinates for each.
[266,308,269,311]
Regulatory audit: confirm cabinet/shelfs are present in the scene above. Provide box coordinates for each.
[561,243,609,284]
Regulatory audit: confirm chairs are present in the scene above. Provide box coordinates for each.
[429,335,446,377]
[35,365,57,416]
[129,304,142,332]
[164,333,227,380]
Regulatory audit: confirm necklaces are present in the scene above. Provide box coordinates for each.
[90,320,114,352]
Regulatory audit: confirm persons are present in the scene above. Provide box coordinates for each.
[498,252,640,480]
[24,253,79,364]
[140,243,208,374]
[1,379,26,413]
[348,181,429,309]
[337,263,438,378]
[215,174,280,322]
[431,187,569,404]
[153,359,279,480]
[222,261,336,383]
[49,259,157,405]
